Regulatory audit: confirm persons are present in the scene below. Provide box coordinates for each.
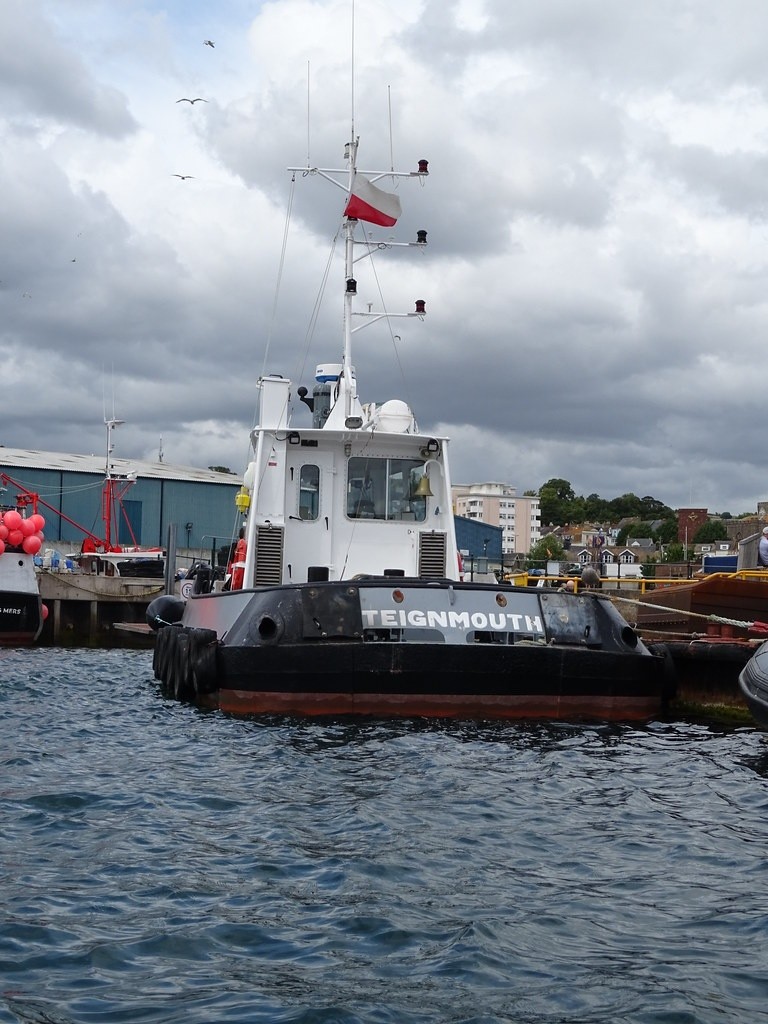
[759,527,768,566]
[459,553,464,581]
[557,583,567,593]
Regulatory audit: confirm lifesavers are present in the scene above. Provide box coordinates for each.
[153,626,222,703]
[457,552,464,581]
[231,538,246,589]
[647,643,678,680]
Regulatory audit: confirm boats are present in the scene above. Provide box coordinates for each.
[737,637,768,725]
[150,0,660,715]
[0,415,231,642]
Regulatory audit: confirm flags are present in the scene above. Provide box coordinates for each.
[344,174,402,227]
